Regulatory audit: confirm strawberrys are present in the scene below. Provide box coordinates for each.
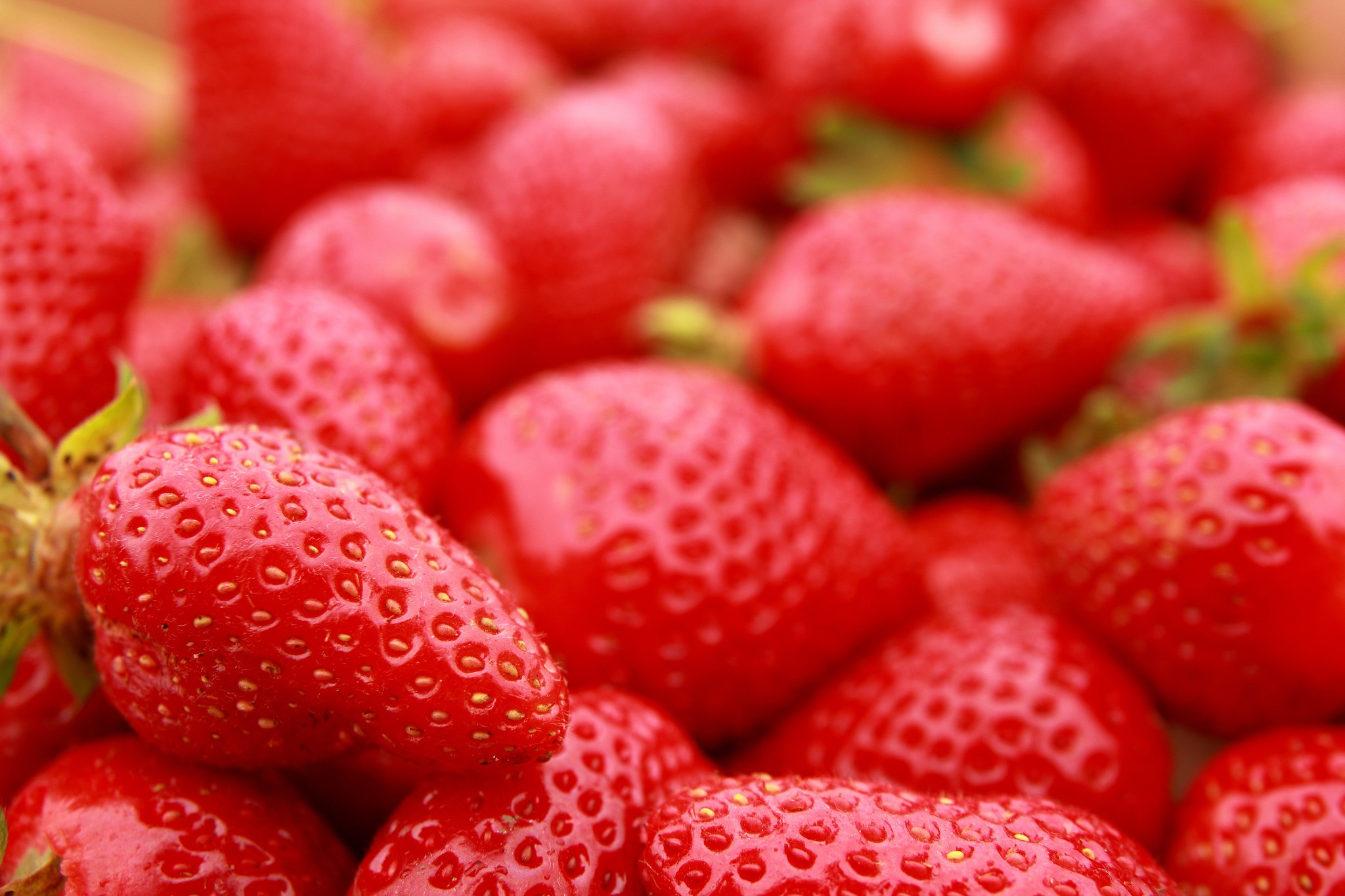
[0,0,1345,896]
